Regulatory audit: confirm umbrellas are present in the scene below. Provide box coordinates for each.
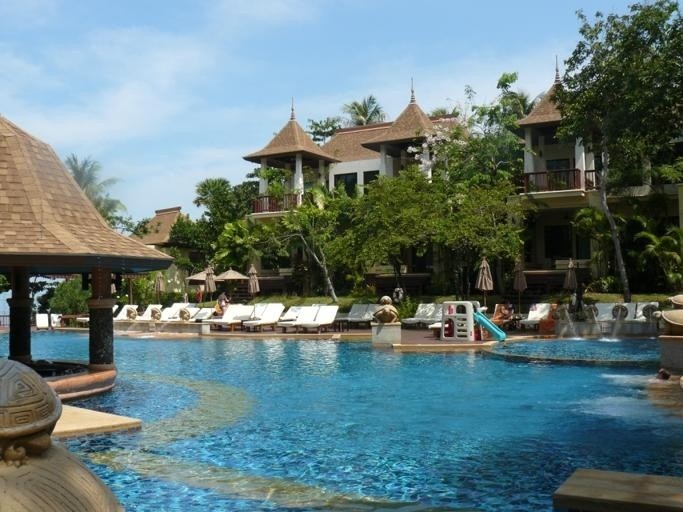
[474,257,493,315]
[205,265,217,302]
[248,264,260,320]
[563,258,577,317]
[155,272,165,304]
[184,268,224,302]
[513,264,527,313]
[215,266,249,304]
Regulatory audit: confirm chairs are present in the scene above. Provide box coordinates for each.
[593,301,659,321]
[75,302,381,335]
[400,302,456,329]
[489,301,569,333]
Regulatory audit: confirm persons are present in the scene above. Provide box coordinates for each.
[218,291,231,314]
[196,287,203,303]
[183,292,188,303]
[495,299,514,321]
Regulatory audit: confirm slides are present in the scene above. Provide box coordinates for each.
[473,311,506,340]
